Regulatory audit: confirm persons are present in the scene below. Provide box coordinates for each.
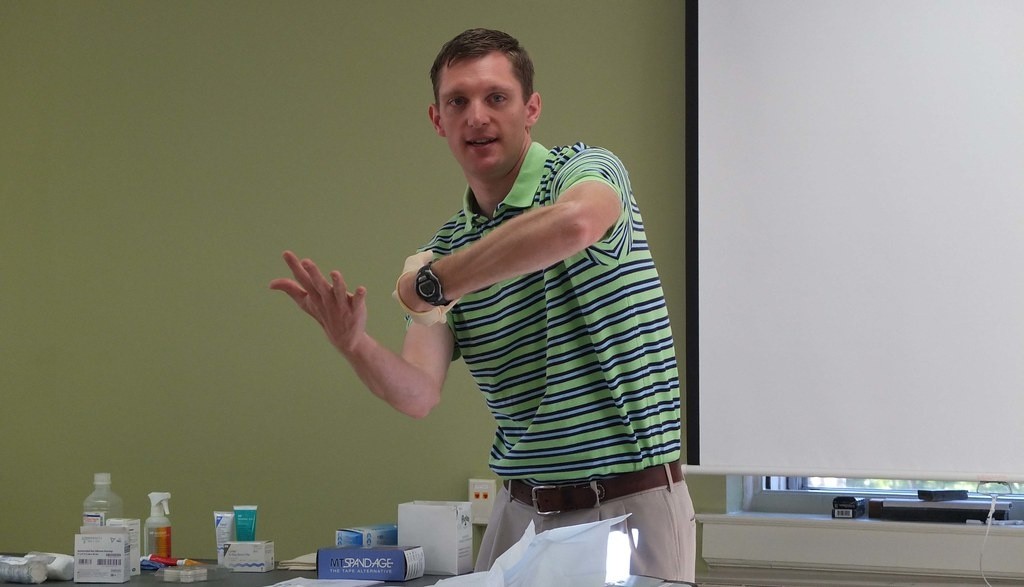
[269,28,698,586]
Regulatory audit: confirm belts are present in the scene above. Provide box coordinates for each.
[501,459,684,516]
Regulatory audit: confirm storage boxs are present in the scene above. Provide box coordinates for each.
[74,518,141,584]
[316,500,474,582]
[222,540,275,573]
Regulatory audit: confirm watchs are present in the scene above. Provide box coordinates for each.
[414,258,455,307]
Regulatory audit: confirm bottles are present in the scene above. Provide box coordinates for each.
[82,472,124,528]
